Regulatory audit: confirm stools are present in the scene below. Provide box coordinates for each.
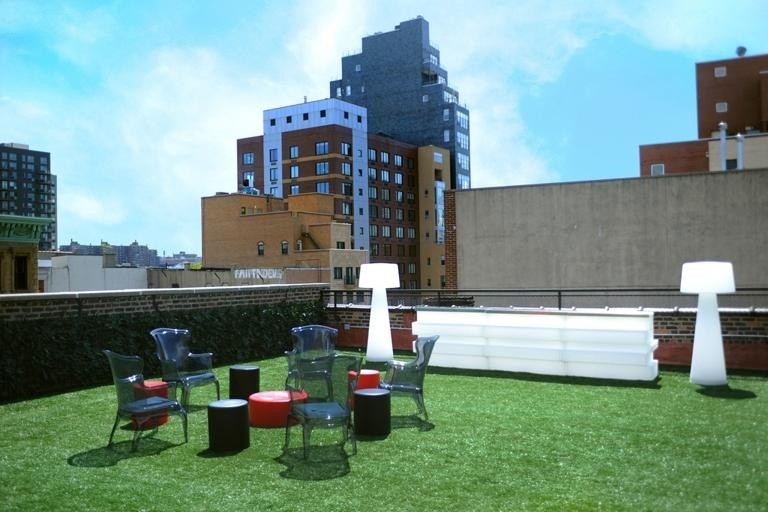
[346,369,392,441]
[207,366,307,452]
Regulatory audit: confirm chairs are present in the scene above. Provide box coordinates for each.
[378,335,441,420]
[101,325,221,452]
[283,323,364,460]
